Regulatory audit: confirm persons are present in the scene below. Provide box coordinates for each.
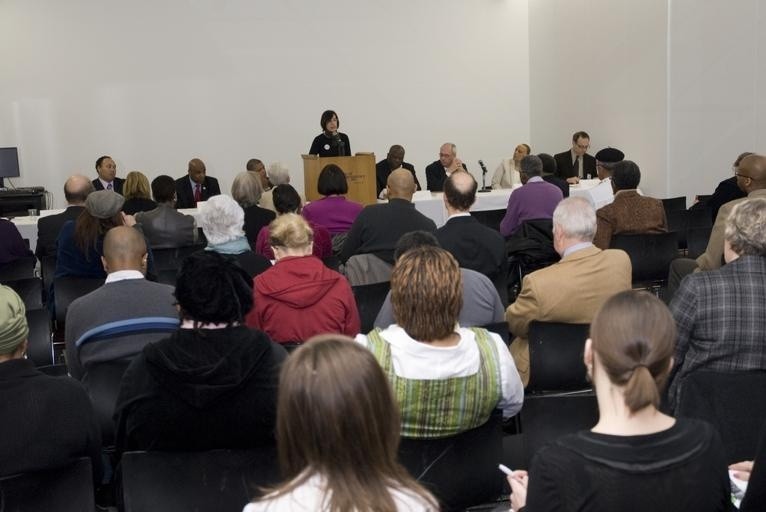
[374,143,764,326]
[353,245,525,441]
[504,197,632,388]
[111,250,289,511]
[552,132,600,186]
[668,189,764,417]
[0,285,102,476]
[1,156,436,369]
[508,289,732,510]
[739,437,765,512]
[241,332,439,511]
[307,110,352,157]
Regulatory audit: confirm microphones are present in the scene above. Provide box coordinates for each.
[478,159,488,173]
[332,130,345,148]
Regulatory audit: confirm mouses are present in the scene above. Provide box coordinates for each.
[32,187,38,193]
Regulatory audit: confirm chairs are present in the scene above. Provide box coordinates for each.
[0,196,766,512]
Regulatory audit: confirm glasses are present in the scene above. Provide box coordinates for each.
[735,171,755,181]
[576,143,590,149]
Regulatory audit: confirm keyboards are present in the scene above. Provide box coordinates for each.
[0,186,33,195]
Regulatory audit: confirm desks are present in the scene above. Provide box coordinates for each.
[12,176,603,252]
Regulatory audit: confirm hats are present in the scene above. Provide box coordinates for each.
[0,284,30,354]
[86,189,126,220]
[595,148,624,162]
[171,248,254,339]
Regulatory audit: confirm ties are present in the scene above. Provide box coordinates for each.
[107,185,112,192]
[194,184,201,208]
[573,156,579,177]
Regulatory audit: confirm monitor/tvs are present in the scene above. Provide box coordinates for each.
[0,147,20,191]
